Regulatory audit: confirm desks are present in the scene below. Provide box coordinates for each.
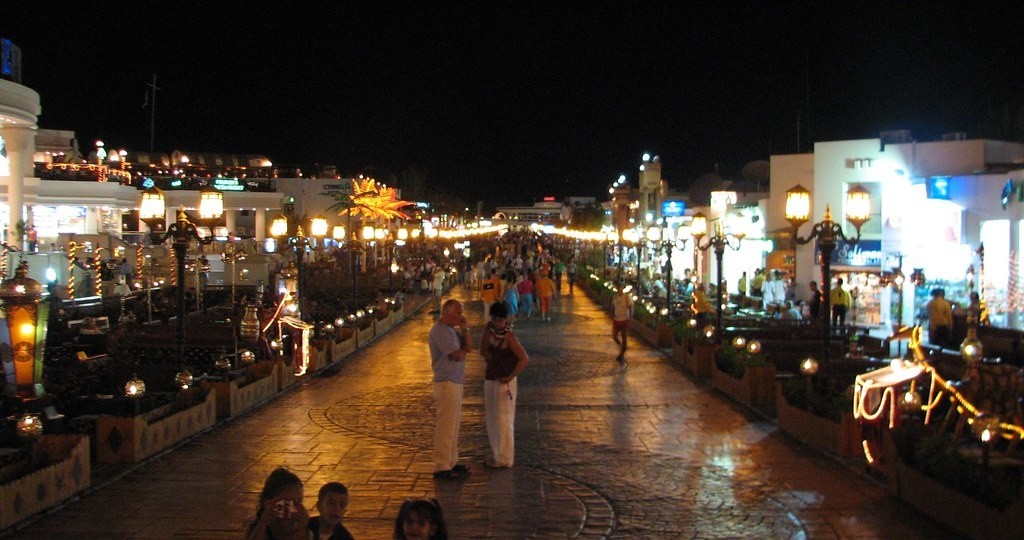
[644,294,1024,459]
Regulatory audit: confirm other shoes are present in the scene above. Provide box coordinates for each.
[435,465,470,480]
[429,310,441,314]
[490,462,512,468]
[617,353,624,361]
[547,317,551,321]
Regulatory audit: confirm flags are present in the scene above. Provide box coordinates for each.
[1001,178,1015,211]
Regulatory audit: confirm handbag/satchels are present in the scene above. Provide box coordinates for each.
[486,331,517,380]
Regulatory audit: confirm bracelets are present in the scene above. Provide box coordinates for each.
[462,328,469,332]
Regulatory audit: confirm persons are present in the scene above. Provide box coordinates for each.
[479,300,529,469]
[229,232,236,245]
[392,497,447,540]
[605,251,988,348]
[28,225,37,254]
[610,281,634,361]
[243,468,355,540]
[38,256,251,334]
[398,228,579,321]
[427,298,473,478]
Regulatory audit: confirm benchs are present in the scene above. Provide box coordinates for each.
[68,316,110,333]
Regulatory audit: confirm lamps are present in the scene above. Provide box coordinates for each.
[958,328,983,366]
[972,413,999,449]
[123,372,145,397]
[0,266,52,402]
[175,369,193,389]
[589,273,762,353]
[897,389,922,415]
[240,305,260,346]
[241,351,255,365]
[800,357,818,375]
[15,415,44,440]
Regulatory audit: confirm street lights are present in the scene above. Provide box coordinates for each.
[269,211,329,322]
[332,209,376,315]
[359,219,691,314]
[777,183,872,368]
[690,209,747,331]
[138,184,225,373]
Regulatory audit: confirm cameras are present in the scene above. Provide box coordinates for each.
[274,500,297,519]
